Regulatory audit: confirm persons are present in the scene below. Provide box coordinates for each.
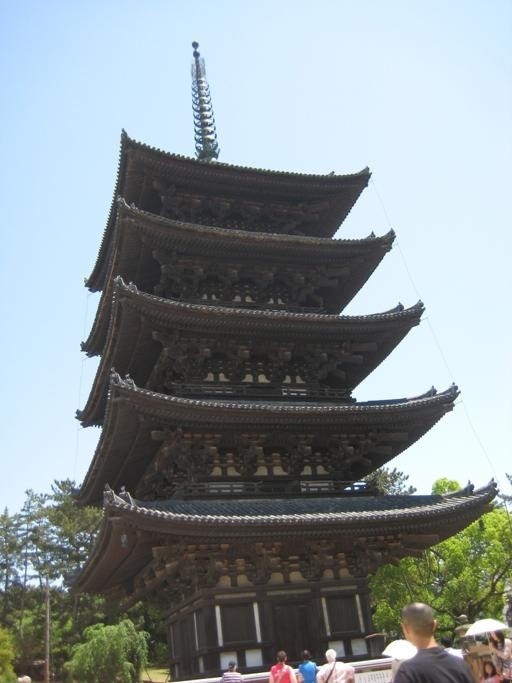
[489,629,512,683]
[316,649,355,683]
[297,650,317,683]
[395,603,475,683]
[481,661,504,683]
[221,661,242,683]
[441,635,463,658]
[269,650,296,683]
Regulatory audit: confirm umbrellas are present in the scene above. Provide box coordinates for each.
[461,619,508,642]
[382,639,417,662]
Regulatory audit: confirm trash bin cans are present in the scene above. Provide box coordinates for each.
[364,633,387,659]
